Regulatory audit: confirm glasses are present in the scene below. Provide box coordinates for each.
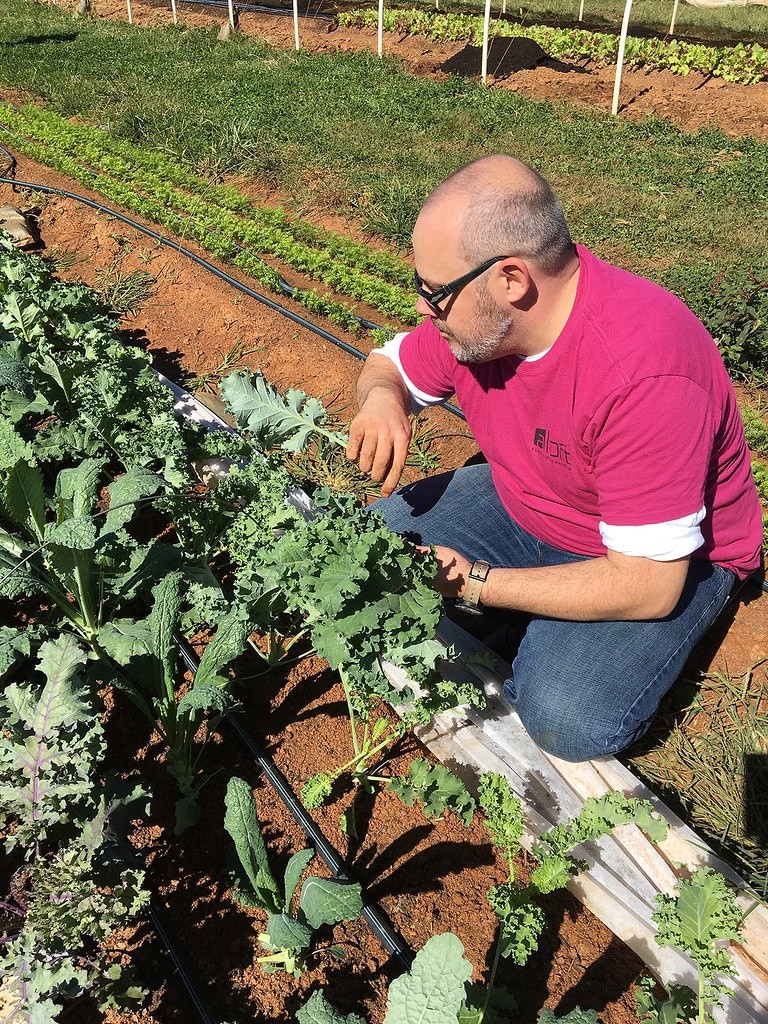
[413,255,514,314]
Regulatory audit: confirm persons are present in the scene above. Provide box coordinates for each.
[345,151,763,762]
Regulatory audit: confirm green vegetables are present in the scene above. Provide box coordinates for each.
[216,364,349,458]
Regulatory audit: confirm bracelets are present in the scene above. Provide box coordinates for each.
[455,559,490,615]
